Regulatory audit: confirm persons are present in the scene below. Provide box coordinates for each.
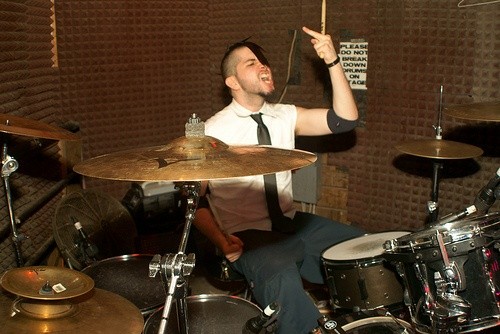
[179,27,367,334]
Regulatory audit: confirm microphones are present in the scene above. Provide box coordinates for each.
[416,205,477,232]
[243,302,279,334]
[71,216,98,258]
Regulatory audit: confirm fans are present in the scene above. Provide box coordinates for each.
[52,188,137,270]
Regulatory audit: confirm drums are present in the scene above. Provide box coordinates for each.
[383,211,500,334]
[79,252,167,322]
[144,294,271,334]
[321,231,413,312]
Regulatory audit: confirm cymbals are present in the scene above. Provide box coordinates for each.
[391,137,483,160]
[72,134,317,181]
[0,113,81,142]
[0,265,95,300]
[1,288,145,334]
[445,101,500,122]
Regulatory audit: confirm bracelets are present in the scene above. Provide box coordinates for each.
[326,57,341,68]
